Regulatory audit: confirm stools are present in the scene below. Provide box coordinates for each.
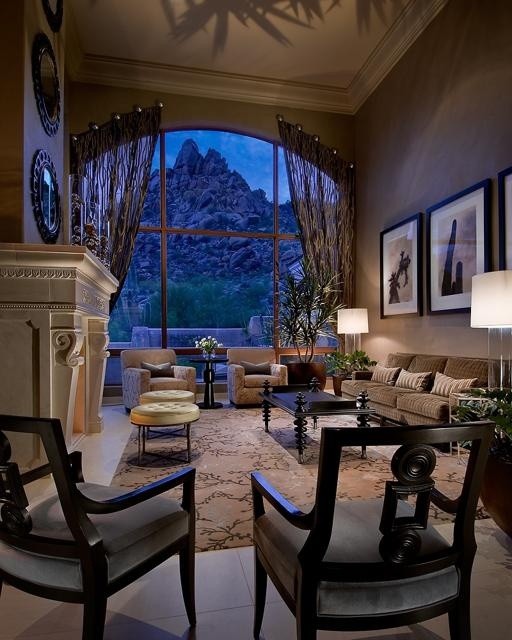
[130,389,200,462]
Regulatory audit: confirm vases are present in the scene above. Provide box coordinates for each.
[203,349,216,360]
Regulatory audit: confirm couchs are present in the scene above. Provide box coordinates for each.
[341,352,505,425]
[121,349,196,413]
[227,348,288,409]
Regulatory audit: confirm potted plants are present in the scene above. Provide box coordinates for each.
[456,399,512,539]
[321,351,376,397]
[275,254,346,392]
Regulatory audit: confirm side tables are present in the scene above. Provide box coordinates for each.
[449,393,512,466]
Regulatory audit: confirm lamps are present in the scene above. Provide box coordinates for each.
[337,308,369,352]
[471,270,512,391]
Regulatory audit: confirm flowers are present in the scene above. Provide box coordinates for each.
[195,336,218,354]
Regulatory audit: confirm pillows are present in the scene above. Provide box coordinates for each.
[141,361,172,377]
[371,362,478,398]
[240,361,271,375]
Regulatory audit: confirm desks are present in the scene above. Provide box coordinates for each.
[189,359,229,409]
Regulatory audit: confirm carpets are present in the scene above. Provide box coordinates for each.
[109,408,492,555]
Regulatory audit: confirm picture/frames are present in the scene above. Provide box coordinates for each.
[379,212,423,319]
[425,178,493,316]
[497,166,512,270]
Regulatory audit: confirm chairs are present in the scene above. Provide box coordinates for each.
[1,415,196,639]
[250,420,496,639]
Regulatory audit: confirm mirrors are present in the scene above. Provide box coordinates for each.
[31,0,66,243]
